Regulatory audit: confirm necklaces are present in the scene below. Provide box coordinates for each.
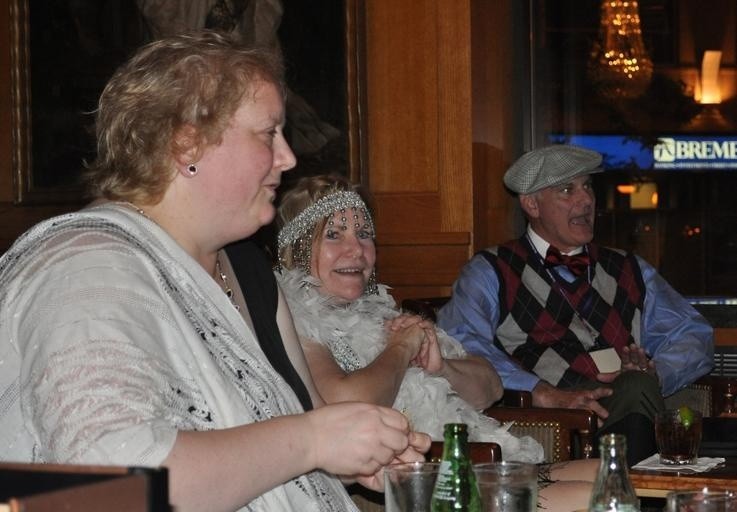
[217,260,240,311]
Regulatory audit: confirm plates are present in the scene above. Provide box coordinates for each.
[632,452,725,476]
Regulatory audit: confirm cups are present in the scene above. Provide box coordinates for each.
[667,487,735,512]
[471,460,538,512]
[383,460,437,511]
[653,409,701,463]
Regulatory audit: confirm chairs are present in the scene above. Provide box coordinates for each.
[402,297,722,460]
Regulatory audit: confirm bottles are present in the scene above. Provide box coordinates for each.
[591,434,641,512]
[719,383,735,419]
[427,422,483,512]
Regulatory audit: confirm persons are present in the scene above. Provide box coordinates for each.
[0,28,432,512]
[274,174,601,512]
[436,145,715,465]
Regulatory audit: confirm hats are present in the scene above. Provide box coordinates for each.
[503,145,605,195]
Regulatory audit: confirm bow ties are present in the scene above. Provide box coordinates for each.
[544,244,589,277]
